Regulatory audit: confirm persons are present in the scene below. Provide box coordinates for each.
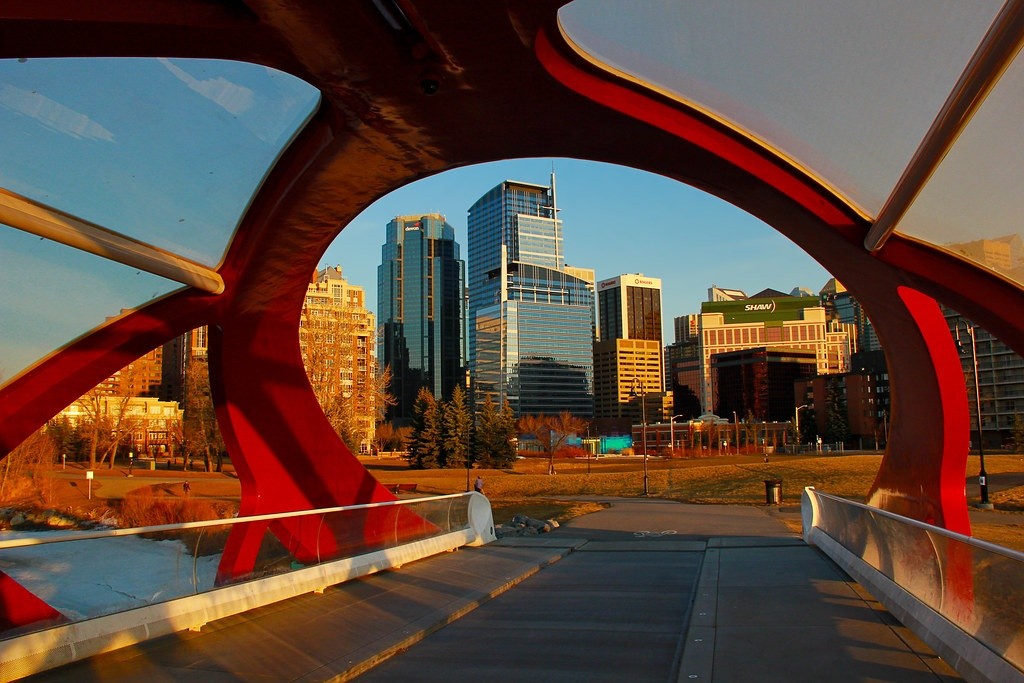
[474,476,484,492]
[183,481,191,494]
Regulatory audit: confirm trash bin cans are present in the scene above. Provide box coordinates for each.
[763,477,783,505]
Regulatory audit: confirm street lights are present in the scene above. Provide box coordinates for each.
[795,405,808,444]
[955,320,995,509]
[670,414,683,451]
[461,427,470,492]
[629,378,650,494]
[733,411,736,424]
